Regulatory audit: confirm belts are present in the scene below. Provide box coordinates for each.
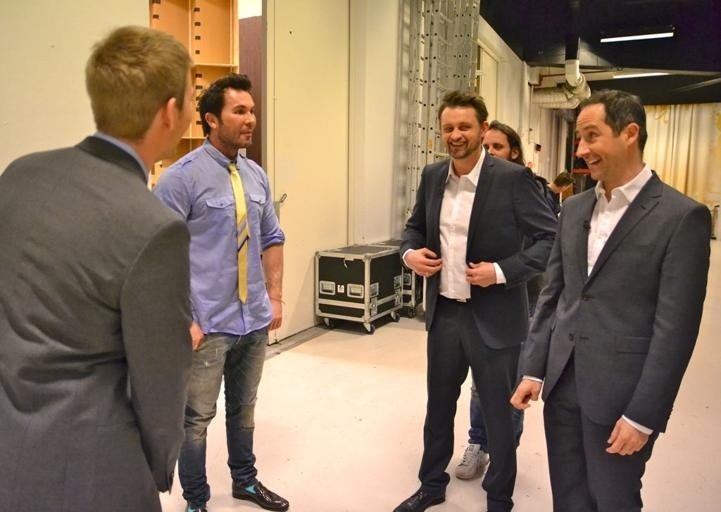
[439,295,470,302]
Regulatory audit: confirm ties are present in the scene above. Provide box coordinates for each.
[228,162,251,302]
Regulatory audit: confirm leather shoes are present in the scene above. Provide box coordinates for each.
[232,480,289,511]
[392,488,445,512]
[185,503,208,512]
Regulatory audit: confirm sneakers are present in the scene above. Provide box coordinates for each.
[456,444,489,478]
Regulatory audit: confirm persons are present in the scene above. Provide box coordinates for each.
[150,74,289,512]
[510,88,711,512]
[547,171,574,219]
[1,25,193,512]
[454,121,556,480]
[396,89,559,512]
